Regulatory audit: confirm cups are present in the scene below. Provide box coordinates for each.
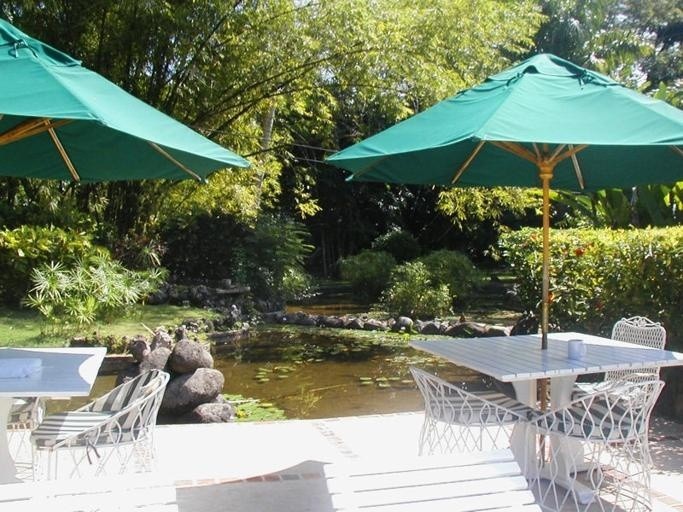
[569,340,586,361]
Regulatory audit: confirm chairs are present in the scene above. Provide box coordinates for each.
[566,315,667,419]
[409,362,529,478]
[7,369,170,512]
[522,377,667,511]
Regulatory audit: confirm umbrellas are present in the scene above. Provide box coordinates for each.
[319,51,681,465]
[0,14,251,191]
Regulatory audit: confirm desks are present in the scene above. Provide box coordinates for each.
[408,333,683,503]
[0,346,107,485]
